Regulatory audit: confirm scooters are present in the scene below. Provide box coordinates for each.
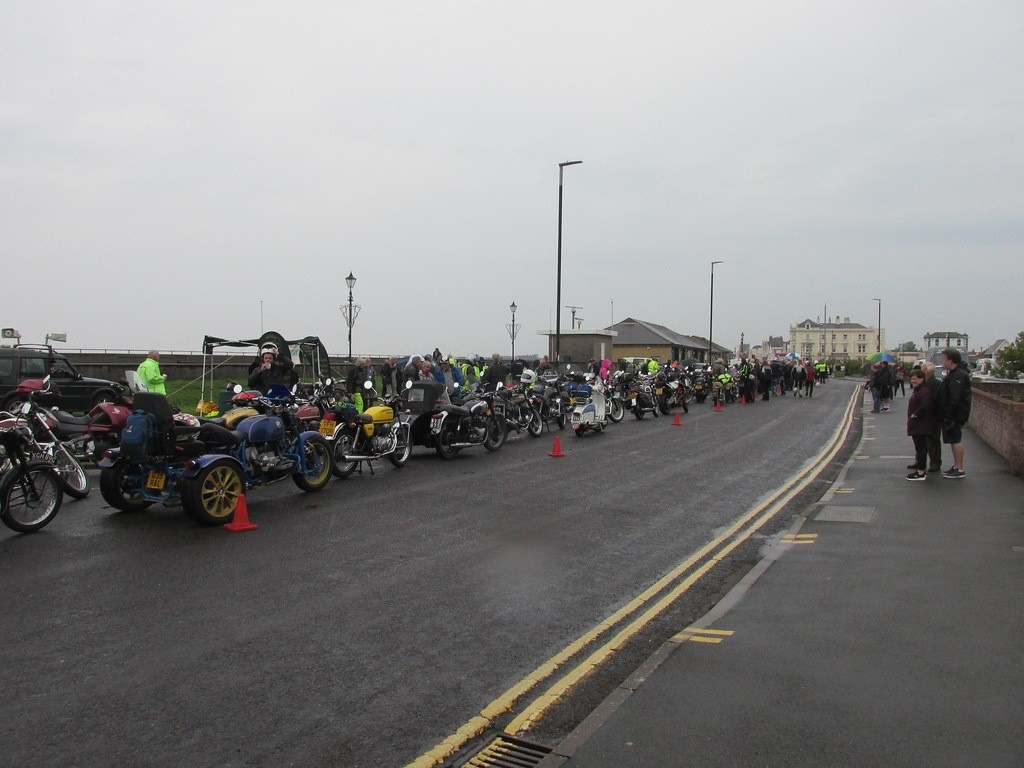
[570,383,608,438]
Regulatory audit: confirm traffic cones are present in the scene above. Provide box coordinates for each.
[672,410,683,425]
[740,395,746,406]
[754,390,758,399]
[713,401,723,412]
[548,435,564,458]
[223,493,259,532]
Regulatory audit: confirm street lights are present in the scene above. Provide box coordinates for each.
[709,261,724,365]
[872,298,880,352]
[505,301,523,359]
[340,271,366,363]
[741,332,744,353]
[556,160,582,367]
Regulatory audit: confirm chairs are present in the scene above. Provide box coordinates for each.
[32,363,44,378]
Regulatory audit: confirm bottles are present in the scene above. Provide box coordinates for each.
[266,363,271,369]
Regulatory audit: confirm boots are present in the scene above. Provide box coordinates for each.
[799,390,802,397]
[793,387,797,396]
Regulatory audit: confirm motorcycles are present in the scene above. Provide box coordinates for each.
[98,375,413,526]
[0,373,135,534]
[388,359,746,461]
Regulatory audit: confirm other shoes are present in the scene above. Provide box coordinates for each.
[881,408,887,410]
[870,410,879,413]
[928,466,940,472]
[908,465,927,469]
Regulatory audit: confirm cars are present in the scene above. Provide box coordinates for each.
[910,358,926,389]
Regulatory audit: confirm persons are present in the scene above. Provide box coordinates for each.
[344,348,841,413]
[248,341,295,396]
[941,348,971,479]
[867,361,941,480]
[134,349,167,397]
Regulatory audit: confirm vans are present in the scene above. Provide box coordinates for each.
[927,346,978,381]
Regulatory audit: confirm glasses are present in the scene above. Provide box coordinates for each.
[441,363,447,364]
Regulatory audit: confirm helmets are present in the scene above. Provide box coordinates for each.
[260,341,279,360]
[520,370,536,383]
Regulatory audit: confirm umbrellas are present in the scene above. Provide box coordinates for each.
[866,352,895,365]
[785,352,801,359]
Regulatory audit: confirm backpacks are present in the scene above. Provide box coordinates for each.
[120,409,160,457]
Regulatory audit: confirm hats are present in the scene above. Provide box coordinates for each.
[439,356,450,363]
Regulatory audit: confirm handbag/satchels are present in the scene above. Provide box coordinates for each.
[864,381,870,390]
[896,372,903,380]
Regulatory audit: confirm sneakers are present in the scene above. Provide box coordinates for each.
[942,465,966,478]
[906,471,927,481]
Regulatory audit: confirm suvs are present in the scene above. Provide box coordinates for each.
[0,344,125,417]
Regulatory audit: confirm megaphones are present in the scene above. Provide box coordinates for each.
[48,333,67,342]
[2,329,20,338]
